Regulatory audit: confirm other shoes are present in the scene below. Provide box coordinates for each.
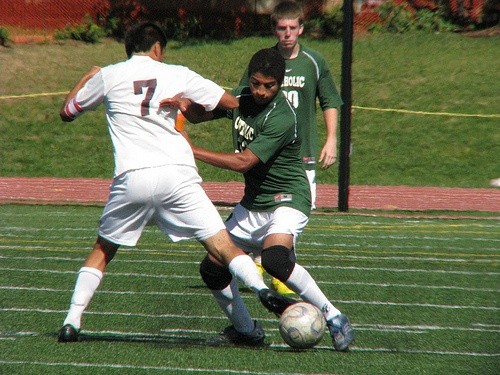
[271,278,295,294]
[253,263,264,277]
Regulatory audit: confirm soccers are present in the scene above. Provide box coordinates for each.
[278,301,326,349]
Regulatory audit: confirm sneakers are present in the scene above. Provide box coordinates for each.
[210,320,265,345]
[54,324,82,342]
[258,288,297,318]
[327,312,353,352]
